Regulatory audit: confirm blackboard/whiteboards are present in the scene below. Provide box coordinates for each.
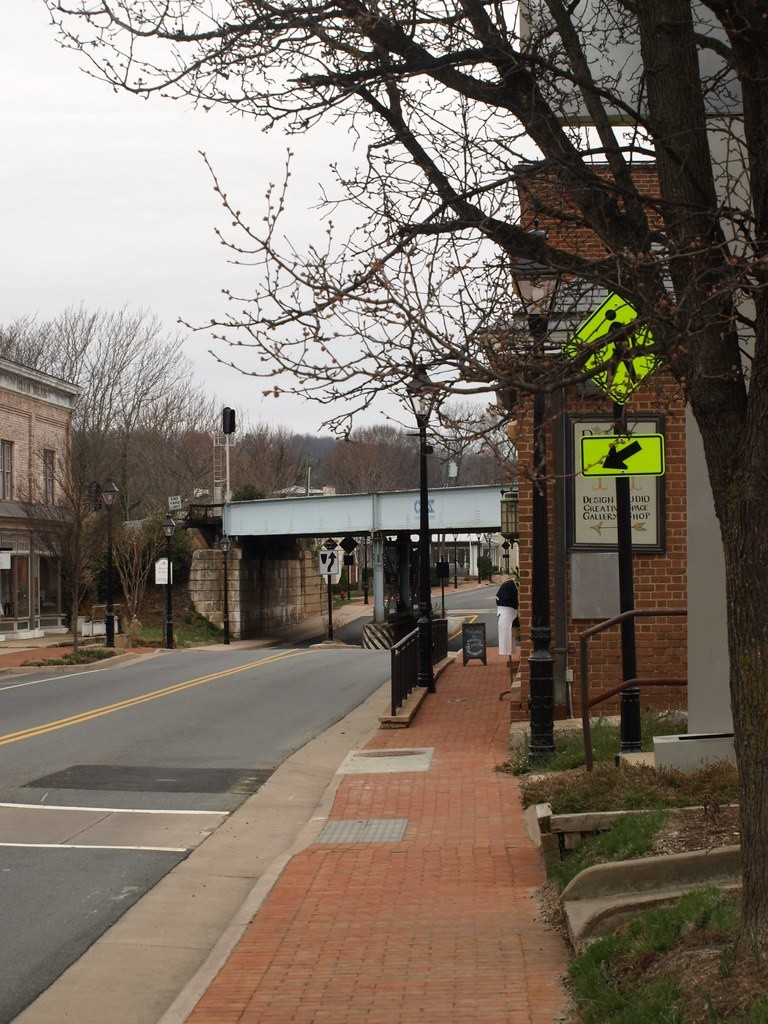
[462,623,486,659]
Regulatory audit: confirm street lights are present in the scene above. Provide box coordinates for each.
[511,216,559,769]
[405,355,441,689]
[486,534,492,581]
[476,532,481,584]
[162,512,179,649]
[453,534,458,588]
[99,476,118,648]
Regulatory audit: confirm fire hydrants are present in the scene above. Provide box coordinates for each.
[339,591,346,600]
